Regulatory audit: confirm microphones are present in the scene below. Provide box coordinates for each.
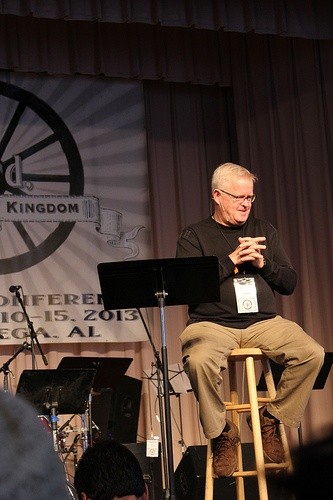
[9,285,21,293]
[58,414,75,432]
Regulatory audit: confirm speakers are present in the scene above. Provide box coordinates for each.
[173,443,275,500]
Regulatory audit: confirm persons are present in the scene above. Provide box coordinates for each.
[75,442,149,500]
[175,163,325,479]
[0,388,70,500]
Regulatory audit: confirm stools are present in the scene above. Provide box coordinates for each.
[204,348,293,500]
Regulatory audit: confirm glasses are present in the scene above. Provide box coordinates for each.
[220,189,256,203]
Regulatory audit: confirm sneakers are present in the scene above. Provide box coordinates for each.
[213,418,241,478]
[247,406,283,463]
